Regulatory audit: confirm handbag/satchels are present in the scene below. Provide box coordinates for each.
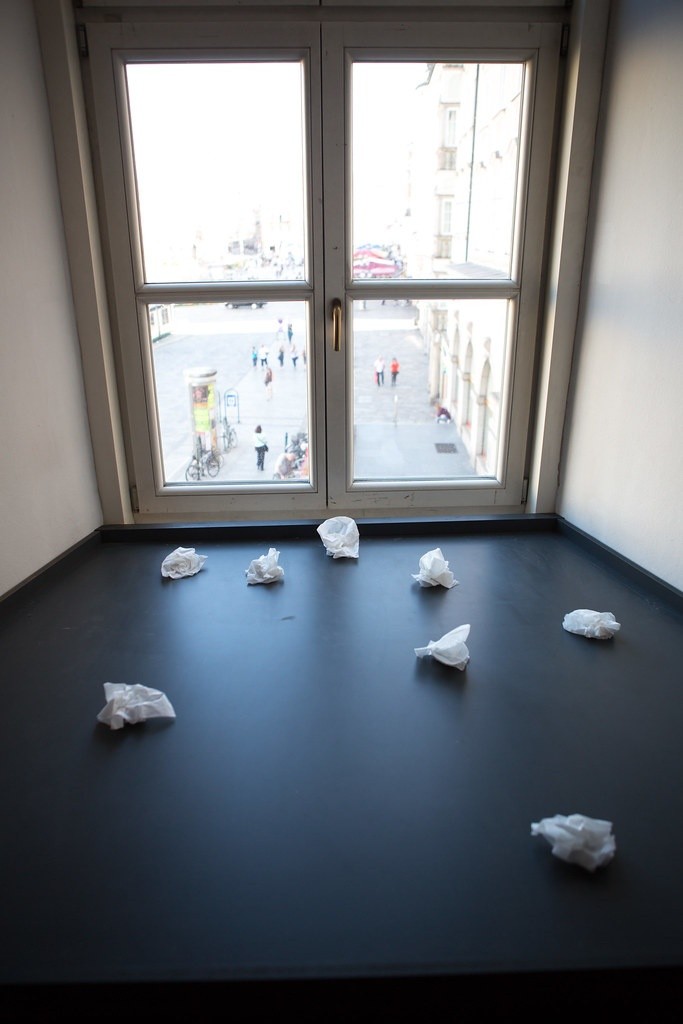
[374,372,378,384]
[262,445,268,452]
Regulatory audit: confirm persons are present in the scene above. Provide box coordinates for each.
[288,320,293,343]
[260,345,268,366]
[252,346,257,366]
[279,346,284,365]
[375,356,385,386]
[290,344,298,367]
[254,426,268,471]
[265,368,272,401]
[390,358,400,388]
[431,402,451,424]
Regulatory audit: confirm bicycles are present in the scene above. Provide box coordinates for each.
[219,424,238,453]
[185,449,220,482]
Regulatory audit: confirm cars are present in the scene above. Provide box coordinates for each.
[225,302,268,310]
